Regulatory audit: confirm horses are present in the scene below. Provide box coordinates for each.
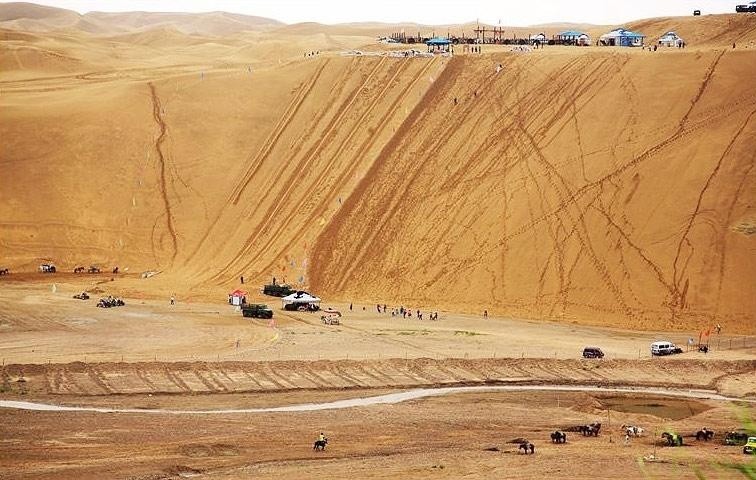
[621,423,645,441]
[519,443,535,454]
[313,438,328,451]
[74,266,85,274]
[551,431,567,444]
[579,423,600,438]
[661,432,683,446]
[695,431,715,442]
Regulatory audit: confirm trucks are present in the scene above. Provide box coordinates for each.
[238,303,273,319]
[263,284,297,298]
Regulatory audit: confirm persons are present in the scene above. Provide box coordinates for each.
[73,291,88,300]
[319,432,324,442]
[170,296,175,305]
[525,441,532,456]
[403,42,737,58]
[484,309,487,317]
[97,295,126,309]
[241,296,246,310]
[583,420,709,448]
[351,303,437,321]
[560,429,564,442]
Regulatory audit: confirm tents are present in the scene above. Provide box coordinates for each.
[281,291,321,312]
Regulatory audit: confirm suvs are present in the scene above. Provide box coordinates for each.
[725,432,747,446]
[743,436,756,455]
[648,341,683,358]
[37,263,57,274]
[323,315,339,326]
[736,1,756,12]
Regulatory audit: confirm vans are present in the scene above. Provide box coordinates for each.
[581,346,607,360]
